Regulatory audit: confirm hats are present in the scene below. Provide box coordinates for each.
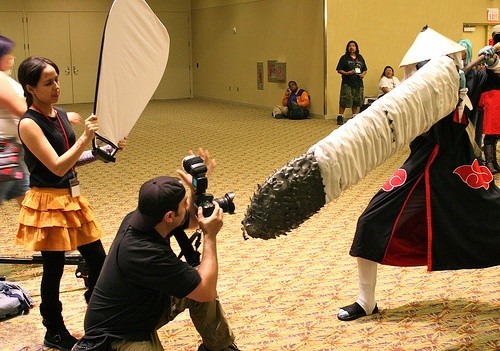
[127,175,187,232]
[399,25,469,69]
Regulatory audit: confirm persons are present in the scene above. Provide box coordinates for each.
[335,40,368,125]
[0,34,84,208]
[377,65,401,99]
[84,147,243,351]
[336,23,500,321]
[276,80,310,120]
[18,56,107,351]
[455,40,475,81]
[472,25,500,174]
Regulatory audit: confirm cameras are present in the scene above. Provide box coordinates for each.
[181,156,236,219]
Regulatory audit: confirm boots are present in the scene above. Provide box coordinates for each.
[38,301,78,350]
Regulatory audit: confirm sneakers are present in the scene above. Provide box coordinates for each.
[197,341,243,351]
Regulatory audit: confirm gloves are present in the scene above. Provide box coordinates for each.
[477,45,496,65]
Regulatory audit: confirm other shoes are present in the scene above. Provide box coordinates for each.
[336,115,343,125]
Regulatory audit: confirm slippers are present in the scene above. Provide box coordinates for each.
[337,302,379,321]
